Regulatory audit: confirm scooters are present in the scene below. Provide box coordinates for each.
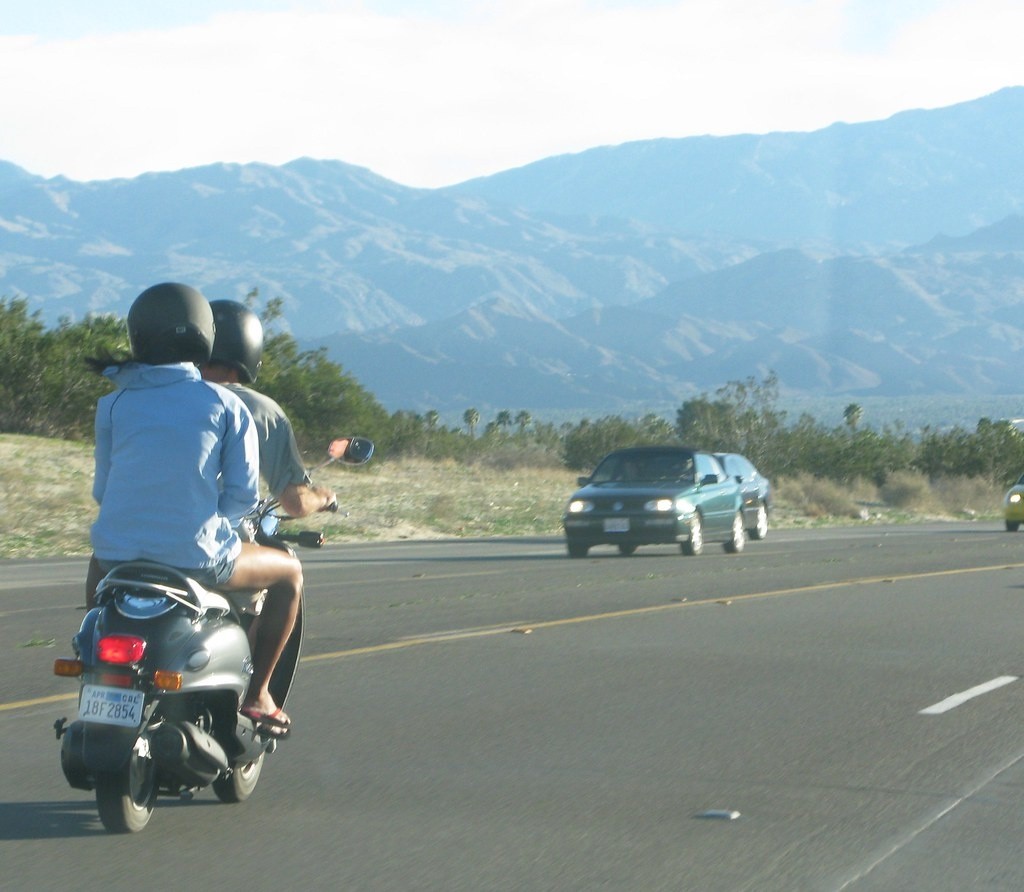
[54,437,375,831]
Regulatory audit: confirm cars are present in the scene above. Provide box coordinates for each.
[655,453,770,528]
[561,448,749,557]
[1003,475,1023,532]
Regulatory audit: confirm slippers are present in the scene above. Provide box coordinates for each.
[240,701,291,729]
[253,718,291,739]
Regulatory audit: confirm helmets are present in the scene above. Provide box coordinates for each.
[127,283,216,368]
[207,299,264,383]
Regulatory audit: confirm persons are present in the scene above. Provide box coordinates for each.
[199,300,337,658]
[666,458,704,482]
[86,284,302,726]
[330,438,372,464]
[616,459,644,480]
